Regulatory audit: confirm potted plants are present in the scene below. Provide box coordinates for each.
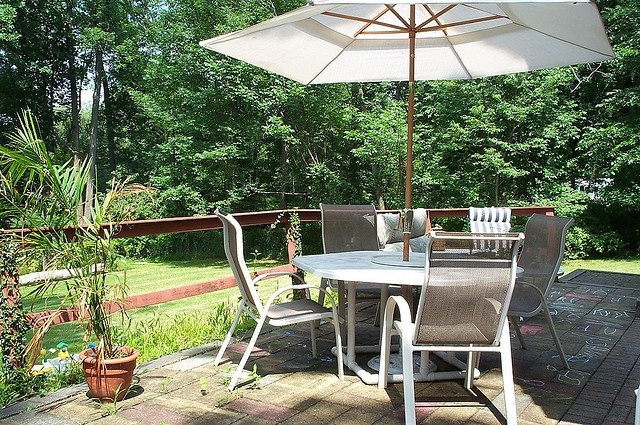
[1,106,156,402]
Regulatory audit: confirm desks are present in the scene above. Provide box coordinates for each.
[293,250,526,385]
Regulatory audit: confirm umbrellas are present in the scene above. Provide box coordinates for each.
[198,1,615,323]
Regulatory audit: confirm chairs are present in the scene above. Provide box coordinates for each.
[468,207,525,321]
[378,231,525,425]
[214,206,344,392]
[314,202,380,345]
[372,208,432,325]
[507,213,575,370]
